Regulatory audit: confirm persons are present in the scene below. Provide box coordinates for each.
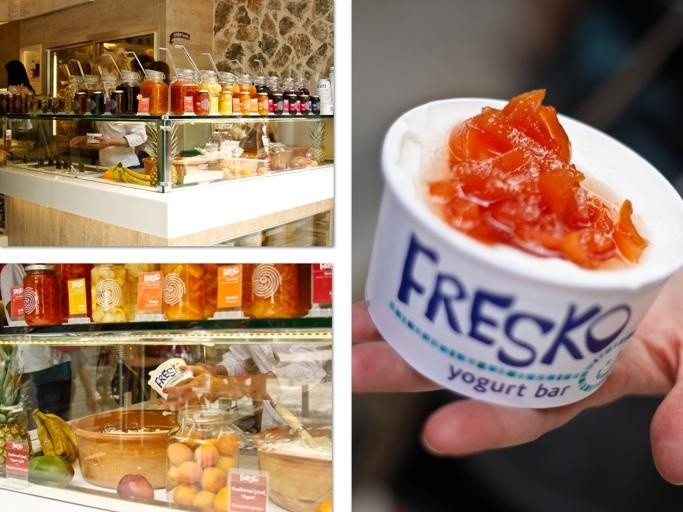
[157,344,333,431]
[1,263,73,425]
[0,136,12,164]
[71,120,147,168]
[5,57,39,139]
[352,266,683,487]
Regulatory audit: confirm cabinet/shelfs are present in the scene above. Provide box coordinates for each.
[0,108,335,248]
[0,324,334,512]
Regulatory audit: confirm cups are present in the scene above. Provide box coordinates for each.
[149,357,193,401]
[86,133,101,144]
[367,96,683,412]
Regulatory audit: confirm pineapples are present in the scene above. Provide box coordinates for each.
[308,147,325,162]
[0,423,32,477]
[151,164,177,187]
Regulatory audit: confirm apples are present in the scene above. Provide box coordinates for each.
[116,474,154,502]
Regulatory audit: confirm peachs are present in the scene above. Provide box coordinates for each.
[165,427,239,512]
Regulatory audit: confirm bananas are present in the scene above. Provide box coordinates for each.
[111,163,151,186]
[102,169,112,181]
[31,407,79,464]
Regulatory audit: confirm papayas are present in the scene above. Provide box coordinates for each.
[29,455,74,487]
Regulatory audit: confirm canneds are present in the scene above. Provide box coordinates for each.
[22,264,311,326]
[0,69,321,117]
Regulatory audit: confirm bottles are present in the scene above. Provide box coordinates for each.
[314,67,335,116]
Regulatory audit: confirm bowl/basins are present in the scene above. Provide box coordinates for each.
[140,143,313,185]
[252,421,331,511]
[69,408,167,491]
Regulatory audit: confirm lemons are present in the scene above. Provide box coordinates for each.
[311,160,317,168]
